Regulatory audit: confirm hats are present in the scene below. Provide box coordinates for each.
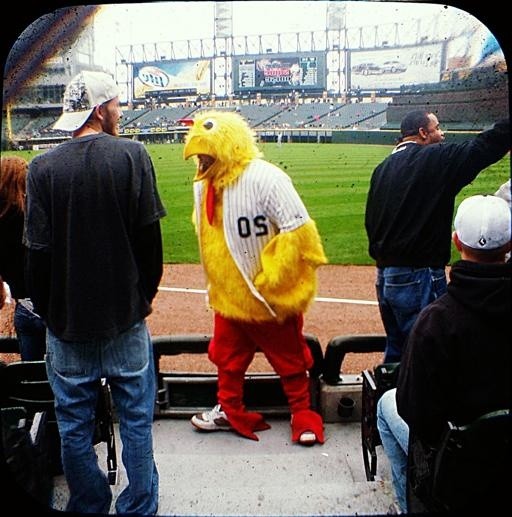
[455,193,511,250]
[51,69,120,133]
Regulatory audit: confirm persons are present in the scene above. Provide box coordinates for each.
[1,155,60,364]
[19,66,168,516]
[362,108,511,365]
[373,190,510,516]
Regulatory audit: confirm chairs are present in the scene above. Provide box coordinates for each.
[361,359,512,517]
[0,358,117,517]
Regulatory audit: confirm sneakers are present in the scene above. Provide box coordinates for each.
[299,430,316,445]
[191,403,234,430]
